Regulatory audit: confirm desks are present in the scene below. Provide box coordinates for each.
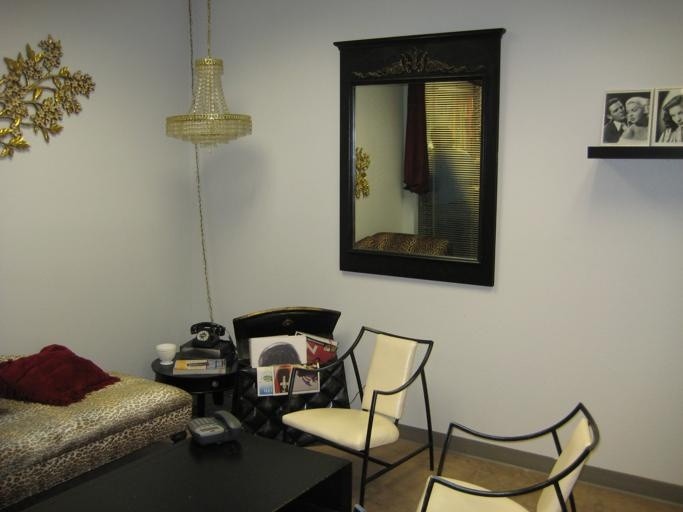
[148,348,237,416]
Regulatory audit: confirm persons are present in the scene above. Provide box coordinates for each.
[417,124,474,239]
[605,96,628,142]
[655,95,682,142]
[621,97,648,144]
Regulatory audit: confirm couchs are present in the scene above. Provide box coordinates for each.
[354,230,451,258]
[0,352,197,507]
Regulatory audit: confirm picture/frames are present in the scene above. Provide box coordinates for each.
[599,84,655,149]
[648,86,683,147]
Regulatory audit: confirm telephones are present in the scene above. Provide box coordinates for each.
[188,410,242,445]
[191,322,225,348]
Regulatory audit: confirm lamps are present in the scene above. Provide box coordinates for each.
[164,2,252,149]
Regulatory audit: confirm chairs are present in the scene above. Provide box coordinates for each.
[413,398,600,512]
[278,323,436,510]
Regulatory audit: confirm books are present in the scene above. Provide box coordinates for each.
[172,357,226,374]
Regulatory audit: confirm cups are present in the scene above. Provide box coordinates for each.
[156,343,176,365]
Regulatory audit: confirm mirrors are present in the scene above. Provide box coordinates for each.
[328,24,508,291]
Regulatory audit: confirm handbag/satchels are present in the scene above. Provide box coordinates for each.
[231,305,348,447]
